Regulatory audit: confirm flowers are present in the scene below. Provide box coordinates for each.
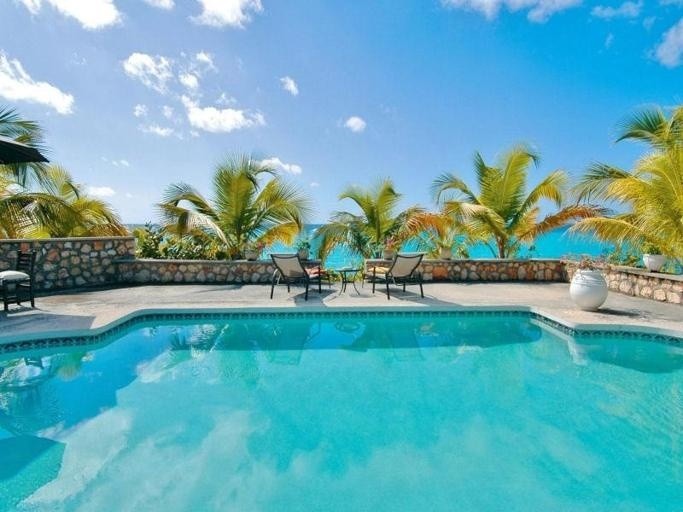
[385,240,394,249]
[255,241,263,252]
[560,254,605,272]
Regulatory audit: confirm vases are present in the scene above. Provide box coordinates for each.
[570,269,607,312]
[383,250,393,260]
[245,249,259,261]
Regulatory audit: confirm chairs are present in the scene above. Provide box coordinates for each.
[270,254,331,301]
[343,325,426,363]
[0,250,36,312]
[24,357,43,370]
[263,320,321,366]
[363,252,424,300]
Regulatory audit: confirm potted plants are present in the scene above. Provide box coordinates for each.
[641,245,667,272]
[437,240,452,261]
[297,239,310,260]
